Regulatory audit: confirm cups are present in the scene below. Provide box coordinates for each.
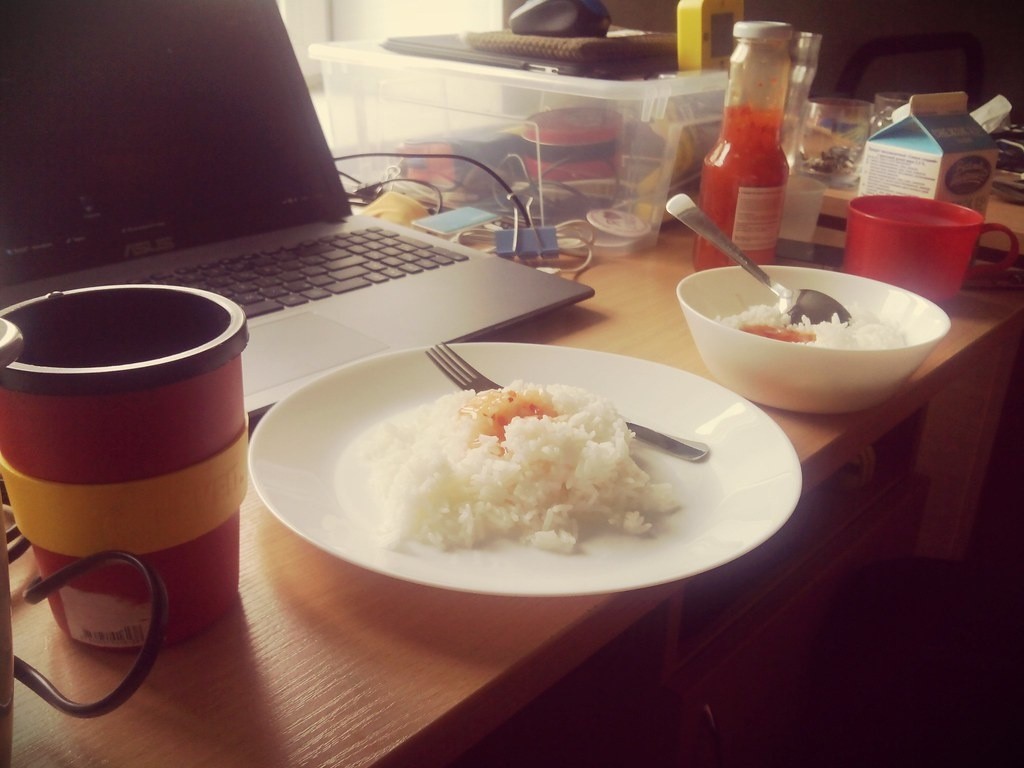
[871,92,909,134]
[842,196,1019,305]
[795,96,874,186]
[776,175,826,243]
[0,284,251,653]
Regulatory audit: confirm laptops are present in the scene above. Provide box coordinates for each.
[0,0,595,420]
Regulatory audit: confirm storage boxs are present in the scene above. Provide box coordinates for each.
[306,37,728,262]
[858,91,999,221]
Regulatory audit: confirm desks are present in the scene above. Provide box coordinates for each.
[0,134,1024,768]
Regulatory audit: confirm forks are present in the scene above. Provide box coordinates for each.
[424,342,710,463]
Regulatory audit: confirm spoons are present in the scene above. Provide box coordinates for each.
[667,193,853,324]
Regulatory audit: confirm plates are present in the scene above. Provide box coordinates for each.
[247,343,803,598]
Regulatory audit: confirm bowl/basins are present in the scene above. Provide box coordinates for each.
[676,265,951,413]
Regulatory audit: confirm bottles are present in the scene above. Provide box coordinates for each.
[693,22,792,277]
